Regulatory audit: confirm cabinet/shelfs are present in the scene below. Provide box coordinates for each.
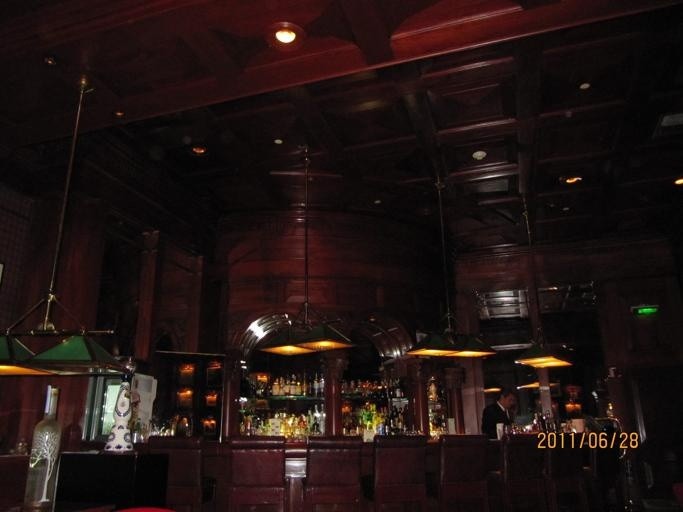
[221,311,428,436]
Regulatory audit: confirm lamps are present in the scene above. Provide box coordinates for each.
[0,73,134,375]
[258,94,357,355]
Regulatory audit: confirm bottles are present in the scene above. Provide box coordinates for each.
[24,385,62,511]
[103,374,135,451]
[237,371,447,442]
[131,418,171,443]
[496,410,572,439]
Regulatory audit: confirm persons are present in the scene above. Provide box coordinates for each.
[480,387,516,439]
